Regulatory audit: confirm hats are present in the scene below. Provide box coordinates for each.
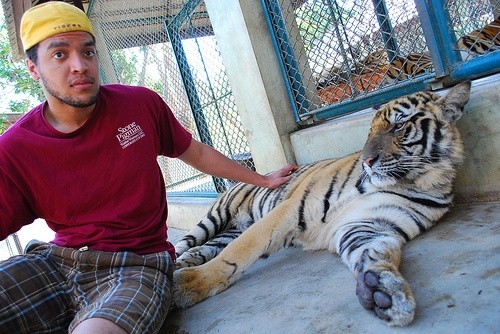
[20,1,96,53]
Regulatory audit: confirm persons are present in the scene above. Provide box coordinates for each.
[0,0,302,334]
